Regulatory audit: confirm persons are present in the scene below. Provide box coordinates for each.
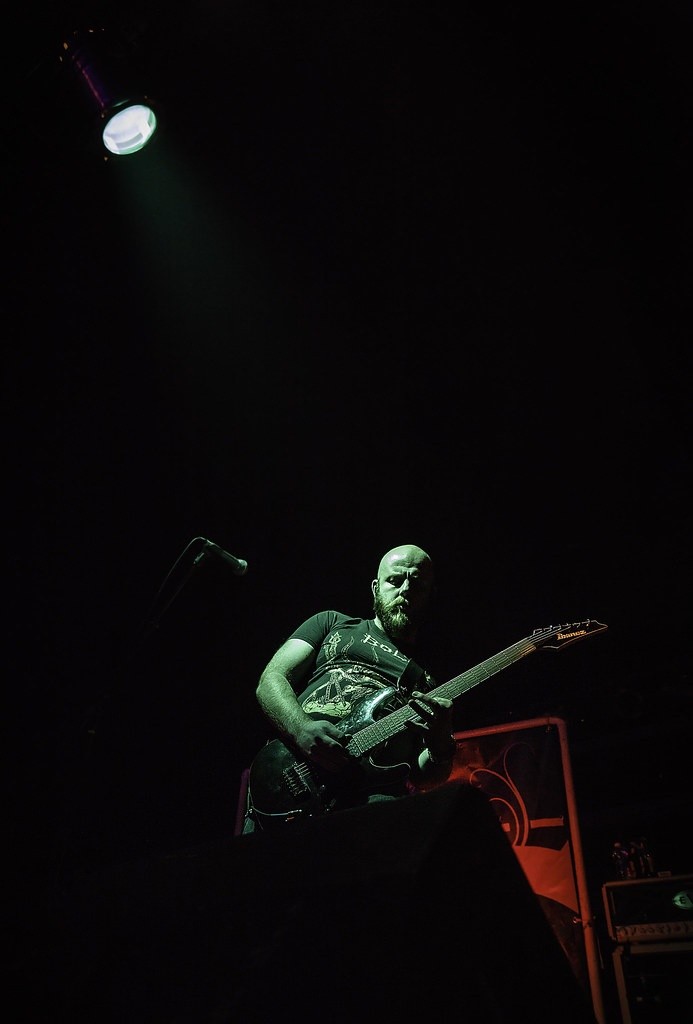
[254,544,458,808]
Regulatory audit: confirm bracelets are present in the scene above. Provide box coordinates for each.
[428,742,458,764]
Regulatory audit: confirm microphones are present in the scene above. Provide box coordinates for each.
[198,536,250,576]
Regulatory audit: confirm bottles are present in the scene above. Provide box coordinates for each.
[611,837,657,881]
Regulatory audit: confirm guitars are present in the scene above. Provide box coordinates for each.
[246,620,609,828]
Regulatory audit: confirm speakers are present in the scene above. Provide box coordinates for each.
[0,783,598,1024]
